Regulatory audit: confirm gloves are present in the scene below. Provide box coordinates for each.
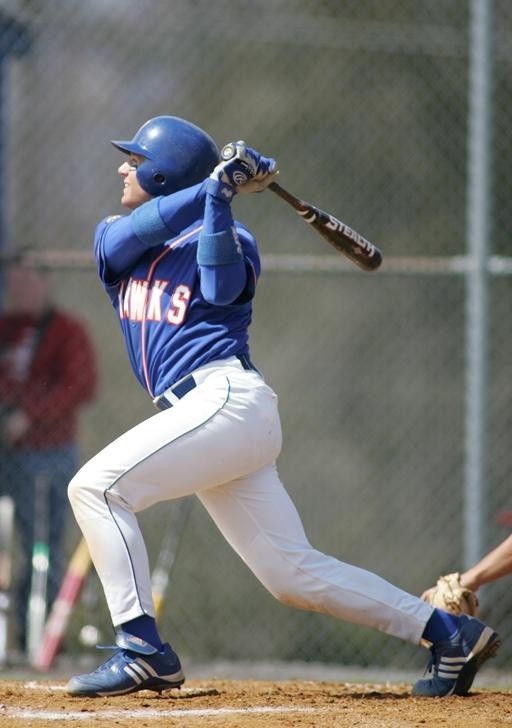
[208,139,279,202]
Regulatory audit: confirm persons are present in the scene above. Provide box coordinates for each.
[420,533,512,650]
[1,254,98,658]
[66,113,500,699]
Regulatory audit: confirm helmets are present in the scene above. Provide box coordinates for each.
[108,115,221,196]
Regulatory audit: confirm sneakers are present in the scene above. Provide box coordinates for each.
[65,630,185,697]
[408,611,499,696]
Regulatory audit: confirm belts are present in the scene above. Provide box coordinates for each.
[152,353,253,410]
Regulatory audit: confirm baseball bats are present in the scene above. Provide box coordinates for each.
[25,474,56,665]
[0,495,17,669]
[220,142,383,273]
[147,490,195,621]
[24,534,95,673]
